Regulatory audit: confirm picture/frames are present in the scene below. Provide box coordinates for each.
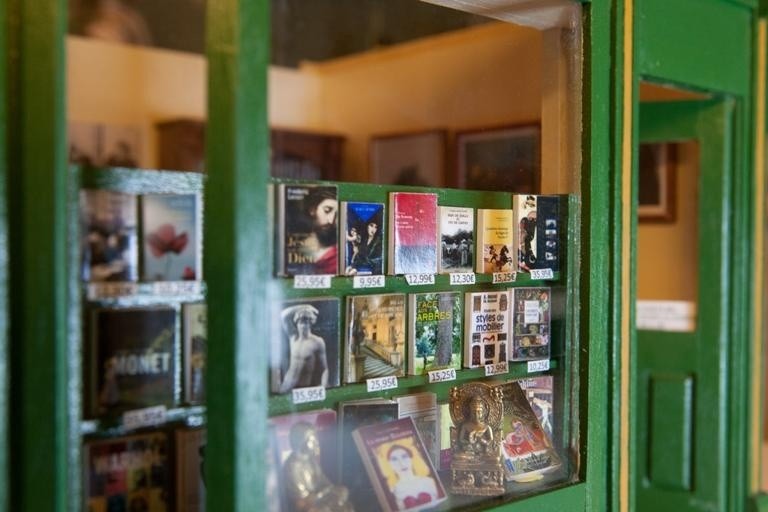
[368,119,541,194]
[636,138,677,224]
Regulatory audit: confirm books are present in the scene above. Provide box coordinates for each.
[270,182,569,511]
[80,192,205,511]
